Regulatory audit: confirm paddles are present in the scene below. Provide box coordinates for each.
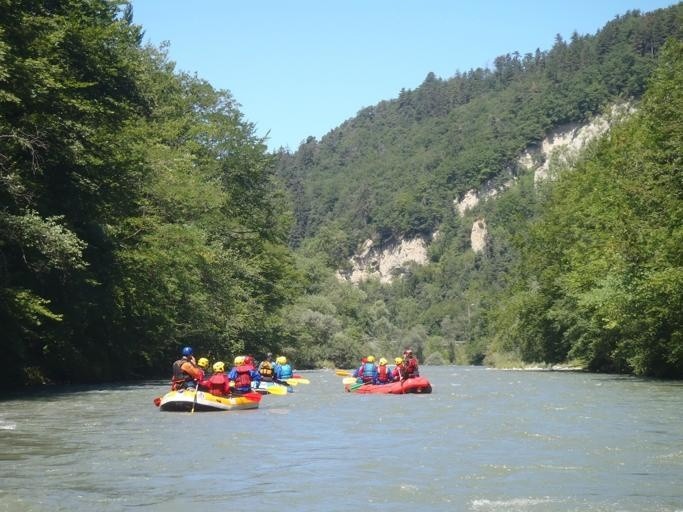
[343,377,372,390]
[154,396,163,406]
[228,377,311,402]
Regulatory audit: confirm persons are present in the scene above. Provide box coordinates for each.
[172,346,419,398]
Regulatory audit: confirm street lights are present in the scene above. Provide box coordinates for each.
[467,302,475,321]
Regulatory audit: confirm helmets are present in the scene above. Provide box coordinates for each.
[395,350,412,365]
[198,357,210,368]
[234,355,253,366]
[263,352,286,364]
[360,355,388,366]
[213,361,226,373]
[181,346,193,358]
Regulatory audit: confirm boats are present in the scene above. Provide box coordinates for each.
[341,376,432,396]
[156,376,311,413]
